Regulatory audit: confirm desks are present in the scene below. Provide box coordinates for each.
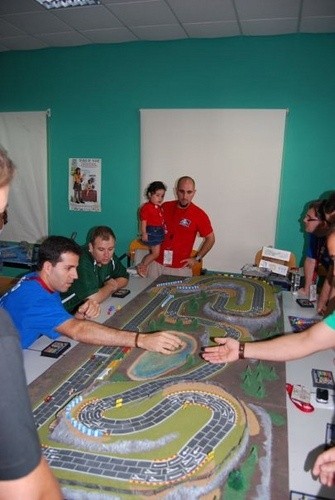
[21,274,335,500]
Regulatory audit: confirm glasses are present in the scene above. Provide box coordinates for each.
[306,213,323,221]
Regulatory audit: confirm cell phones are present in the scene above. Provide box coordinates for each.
[316,388,328,402]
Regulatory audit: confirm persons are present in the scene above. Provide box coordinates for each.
[0,236,185,354]
[0,146,61,500]
[59,225,129,316]
[297,191,335,317]
[202,230,335,364]
[147,176,215,277]
[312,447,335,487]
[133,181,168,277]
[72,167,85,204]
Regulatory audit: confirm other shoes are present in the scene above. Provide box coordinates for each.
[138,263,148,278]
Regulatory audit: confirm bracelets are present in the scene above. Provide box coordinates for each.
[238,342,245,359]
[195,255,201,262]
[135,333,139,347]
